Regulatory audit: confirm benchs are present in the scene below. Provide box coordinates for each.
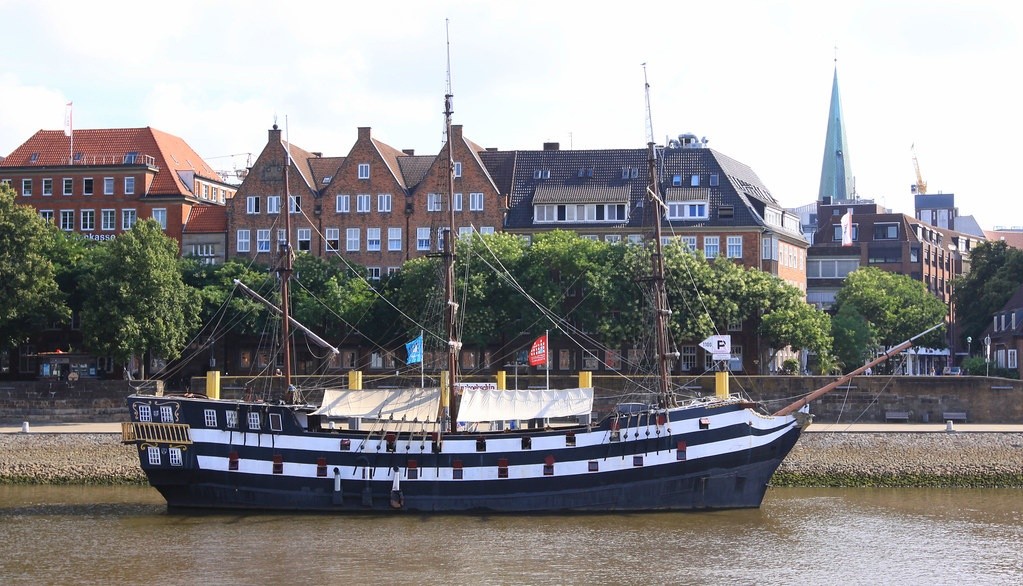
[943,412,967,424]
[885,411,909,424]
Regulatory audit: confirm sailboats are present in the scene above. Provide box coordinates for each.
[120,17,816,517]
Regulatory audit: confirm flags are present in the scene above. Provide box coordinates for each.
[840,210,853,248]
[528,334,547,366]
[404,335,422,365]
